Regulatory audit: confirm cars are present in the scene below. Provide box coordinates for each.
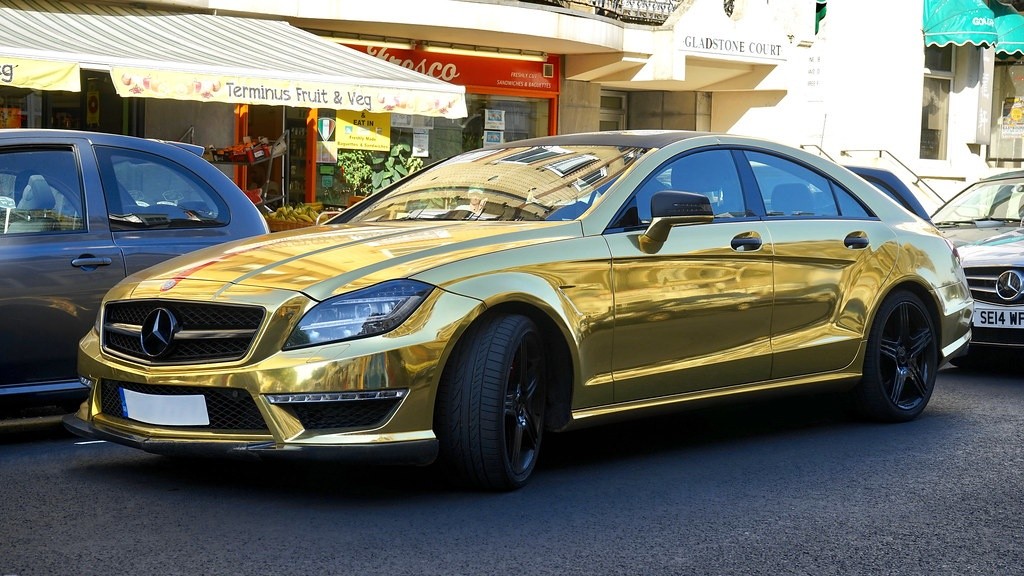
[0,126,270,424]
[753,166,936,230]
[928,169,1024,368]
[61,127,976,491]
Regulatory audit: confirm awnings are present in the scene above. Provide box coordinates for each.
[923,0,998,49]
[0,2,468,120]
[989,0,1024,55]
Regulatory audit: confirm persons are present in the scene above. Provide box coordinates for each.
[5,170,44,215]
[454,184,485,220]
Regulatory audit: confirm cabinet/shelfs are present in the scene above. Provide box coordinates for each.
[177,126,289,214]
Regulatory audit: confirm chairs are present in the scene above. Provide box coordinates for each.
[770,183,816,215]
[5,171,60,233]
[669,153,723,214]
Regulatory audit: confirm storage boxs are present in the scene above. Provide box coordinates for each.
[202,141,286,164]
[263,213,336,233]
